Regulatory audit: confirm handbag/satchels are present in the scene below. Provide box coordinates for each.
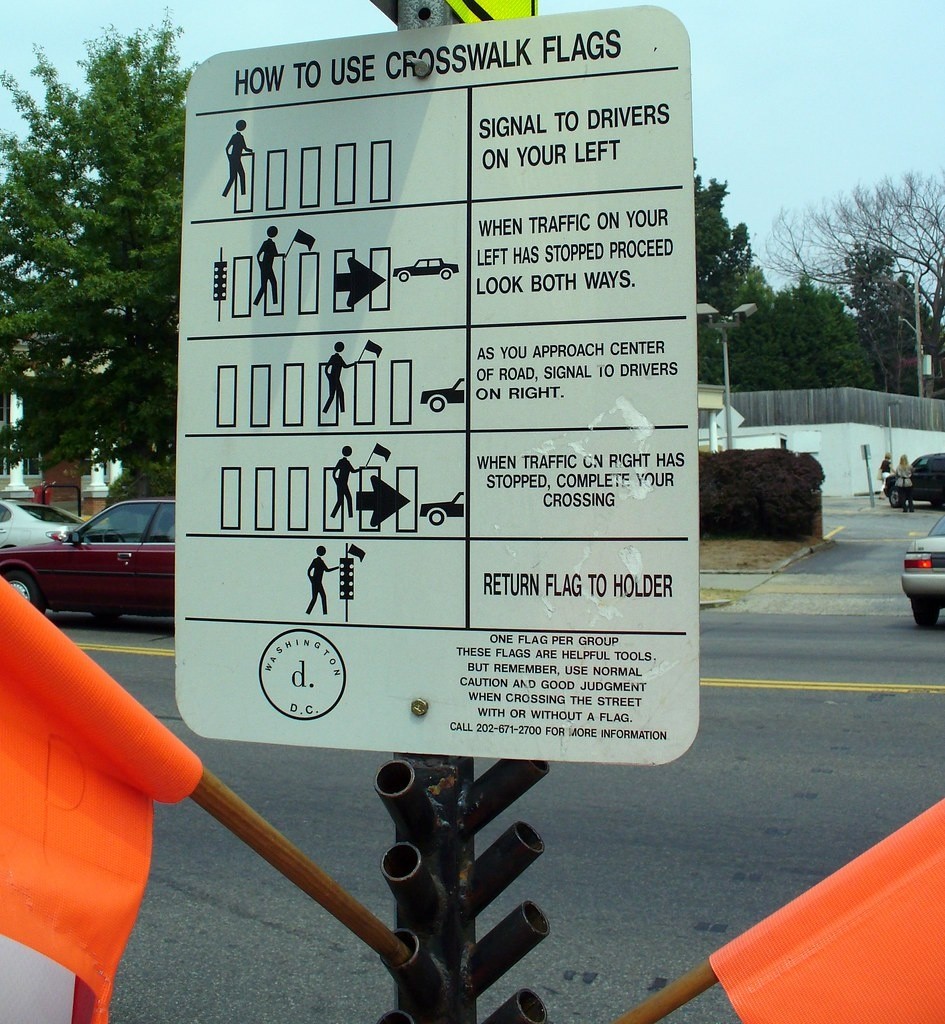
[877,468,883,479]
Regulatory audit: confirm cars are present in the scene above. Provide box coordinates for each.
[0,496,178,624]
[884,453,945,509]
[900,509,945,626]
[0,500,89,546]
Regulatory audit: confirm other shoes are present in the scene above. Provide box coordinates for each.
[903,510,908,512]
[909,510,913,512]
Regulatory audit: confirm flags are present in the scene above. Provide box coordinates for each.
[709,797,945,1024]
[0,577,203,1024]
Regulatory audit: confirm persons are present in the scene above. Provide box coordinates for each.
[896,455,915,512]
[878,453,896,500]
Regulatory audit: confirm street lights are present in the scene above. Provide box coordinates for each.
[898,316,924,397]
[697,303,757,451]
